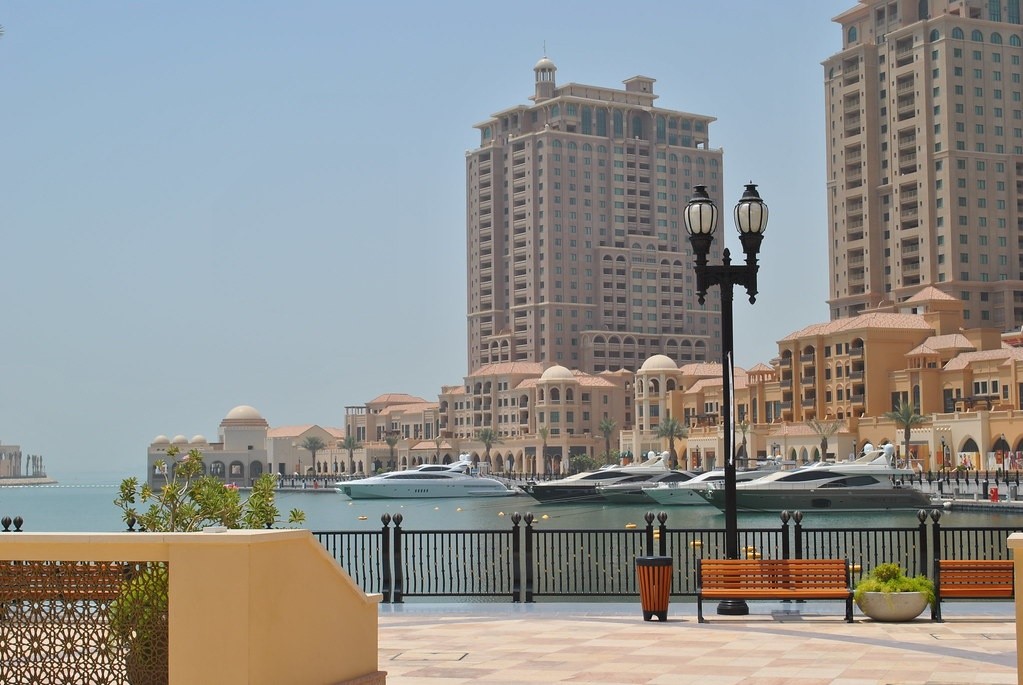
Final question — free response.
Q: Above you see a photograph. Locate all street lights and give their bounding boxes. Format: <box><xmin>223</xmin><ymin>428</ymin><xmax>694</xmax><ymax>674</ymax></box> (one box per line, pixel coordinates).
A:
<box><xmin>772</xmin><ymin>441</ymin><xmax>777</xmax><ymax>466</ymax></box>
<box><xmin>1001</xmin><ymin>433</ymin><xmax>1006</xmax><ymax>482</ymax></box>
<box><xmin>681</xmin><ymin>179</ymin><xmax>770</xmax><ymax>612</ymax></box>
<box><xmin>940</xmin><ymin>435</ymin><xmax>945</xmax><ymax>480</ymax></box>
<box><xmin>853</xmin><ymin>440</ymin><xmax>856</xmax><ymax>460</ymax></box>
<box><xmin>695</xmin><ymin>445</ymin><xmax>699</xmax><ymax>468</ymax></box>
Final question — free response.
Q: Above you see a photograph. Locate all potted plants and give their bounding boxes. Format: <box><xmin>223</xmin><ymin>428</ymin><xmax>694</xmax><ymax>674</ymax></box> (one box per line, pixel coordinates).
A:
<box><xmin>854</xmin><ymin>564</ymin><xmax>934</xmax><ymax>621</ymax></box>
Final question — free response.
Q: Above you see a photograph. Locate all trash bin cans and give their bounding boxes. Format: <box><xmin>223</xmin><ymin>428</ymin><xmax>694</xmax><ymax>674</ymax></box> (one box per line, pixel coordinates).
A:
<box><xmin>636</xmin><ymin>556</ymin><xmax>673</xmax><ymax>622</ymax></box>
<box><xmin>1010</xmin><ymin>486</ymin><xmax>1017</xmax><ymax>500</ymax></box>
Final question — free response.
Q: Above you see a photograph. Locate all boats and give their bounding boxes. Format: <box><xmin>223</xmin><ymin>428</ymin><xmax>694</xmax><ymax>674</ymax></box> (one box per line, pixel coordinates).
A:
<box><xmin>333</xmin><ymin>451</ymin><xmax>519</xmax><ymax>501</ymax></box>
<box><xmin>514</xmin><ymin>440</ymin><xmax>953</xmax><ymax>515</ymax></box>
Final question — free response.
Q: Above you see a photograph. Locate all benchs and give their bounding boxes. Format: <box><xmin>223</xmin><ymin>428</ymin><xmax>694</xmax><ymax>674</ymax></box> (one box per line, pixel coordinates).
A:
<box><xmin>695</xmin><ymin>558</ymin><xmax>854</xmax><ymax>623</ymax></box>
<box><xmin>933</xmin><ymin>558</ymin><xmax>1015</xmax><ymax>623</ymax></box>
<box><xmin>0</xmin><ymin>563</ymin><xmax>128</xmax><ymax>611</ymax></box>
